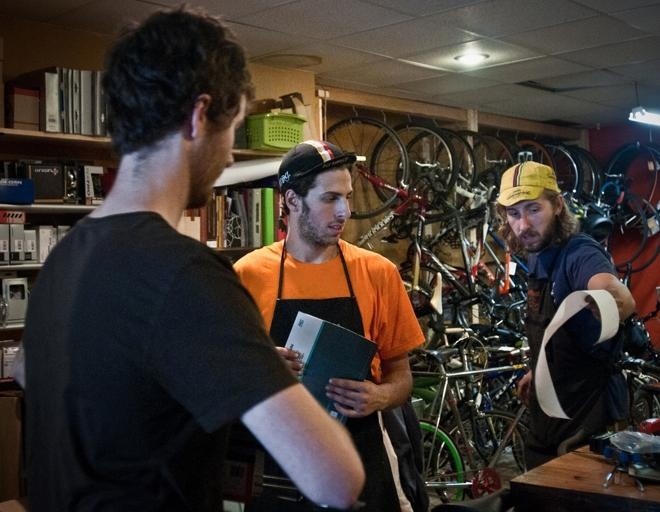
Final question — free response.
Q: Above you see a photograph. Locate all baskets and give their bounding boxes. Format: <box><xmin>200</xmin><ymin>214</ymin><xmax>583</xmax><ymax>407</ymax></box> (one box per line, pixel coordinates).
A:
<box><xmin>237</xmin><ymin>114</ymin><xmax>308</xmax><ymax>150</ymax></box>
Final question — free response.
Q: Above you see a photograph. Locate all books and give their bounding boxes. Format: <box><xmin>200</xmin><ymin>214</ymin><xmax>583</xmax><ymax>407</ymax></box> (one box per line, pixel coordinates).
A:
<box><xmin>31</xmin><ymin>65</ymin><xmax>112</xmax><ymax>138</ymax></box>
<box><xmin>280</xmin><ymin>308</ymin><xmax>380</xmax><ymax>439</ymax></box>
<box><xmin>181</xmin><ymin>185</ymin><xmax>291</xmax><ymax>250</ymax></box>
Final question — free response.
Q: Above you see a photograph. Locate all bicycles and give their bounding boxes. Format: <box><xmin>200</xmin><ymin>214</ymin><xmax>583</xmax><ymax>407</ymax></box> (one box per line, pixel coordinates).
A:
<box><xmin>326</xmin><ymin>115</ymin><xmax>660</xmax><ymax>503</ymax></box>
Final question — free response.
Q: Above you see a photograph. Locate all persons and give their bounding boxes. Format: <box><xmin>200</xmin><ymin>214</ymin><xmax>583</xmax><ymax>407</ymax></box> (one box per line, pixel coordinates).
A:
<box><xmin>229</xmin><ymin>139</ymin><xmax>428</xmax><ymax>510</ymax></box>
<box><xmin>19</xmin><ymin>7</ymin><xmax>372</xmax><ymax>512</ymax></box>
<box><xmin>493</xmin><ymin>159</ymin><xmax>638</xmax><ymax>476</ymax></box>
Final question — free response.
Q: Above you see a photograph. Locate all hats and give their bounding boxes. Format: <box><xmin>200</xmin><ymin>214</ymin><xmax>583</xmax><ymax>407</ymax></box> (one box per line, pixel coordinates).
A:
<box><xmin>497</xmin><ymin>160</ymin><xmax>561</xmax><ymax>207</ymax></box>
<box><xmin>279</xmin><ymin>139</ymin><xmax>358</xmax><ymax>193</ymax></box>
